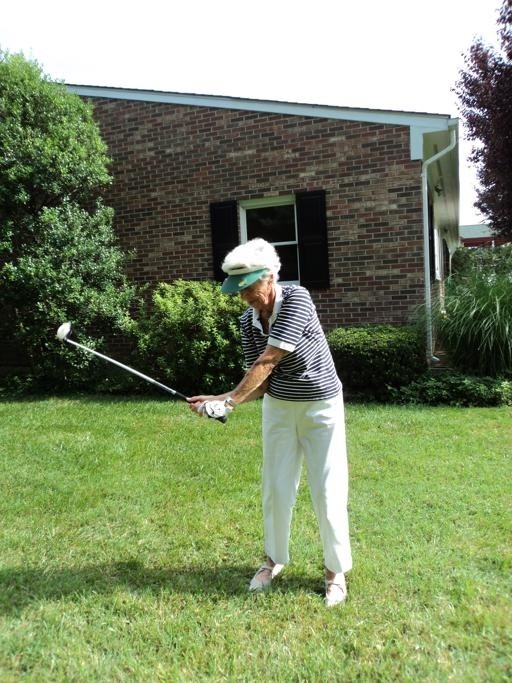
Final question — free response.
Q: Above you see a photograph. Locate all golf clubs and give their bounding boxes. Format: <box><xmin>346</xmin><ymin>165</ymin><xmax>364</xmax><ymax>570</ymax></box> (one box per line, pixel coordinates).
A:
<box><xmin>56</xmin><ymin>321</ymin><xmax>227</xmax><ymax>423</ymax></box>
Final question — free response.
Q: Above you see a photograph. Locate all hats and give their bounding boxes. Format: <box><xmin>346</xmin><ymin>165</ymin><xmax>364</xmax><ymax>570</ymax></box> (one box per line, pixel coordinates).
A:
<box><xmin>219</xmin><ymin>237</ymin><xmax>281</xmax><ymax>294</ymax></box>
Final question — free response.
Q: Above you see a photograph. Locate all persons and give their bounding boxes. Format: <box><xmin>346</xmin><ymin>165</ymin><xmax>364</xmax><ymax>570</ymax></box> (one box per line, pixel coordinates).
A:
<box><xmin>184</xmin><ymin>234</ymin><xmax>357</xmax><ymax>608</ymax></box>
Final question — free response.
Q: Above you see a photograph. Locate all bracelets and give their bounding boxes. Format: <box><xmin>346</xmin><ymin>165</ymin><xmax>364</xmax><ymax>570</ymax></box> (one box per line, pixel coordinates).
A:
<box><xmin>225</xmin><ymin>395</ymin><xmax>237</xmax><ymax>408</ymax></box>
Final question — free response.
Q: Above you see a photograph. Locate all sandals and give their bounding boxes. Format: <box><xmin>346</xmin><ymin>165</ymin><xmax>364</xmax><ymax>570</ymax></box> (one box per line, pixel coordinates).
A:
<box><xmin>324</xmin><ymin>567</ymin><xmax>347</xmax><ymax>608</ymax></box>
<box><xmin>248</xmin><ymin>564</ymin><xmax>274</xmax><ymax>592</ymax></box>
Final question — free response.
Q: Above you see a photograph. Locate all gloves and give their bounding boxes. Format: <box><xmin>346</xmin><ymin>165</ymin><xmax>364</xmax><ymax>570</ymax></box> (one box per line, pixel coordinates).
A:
<box><xmin>197</xmin><ymin>399</ymin><xmax>233</xmax><ymax>420</ymax></box>
